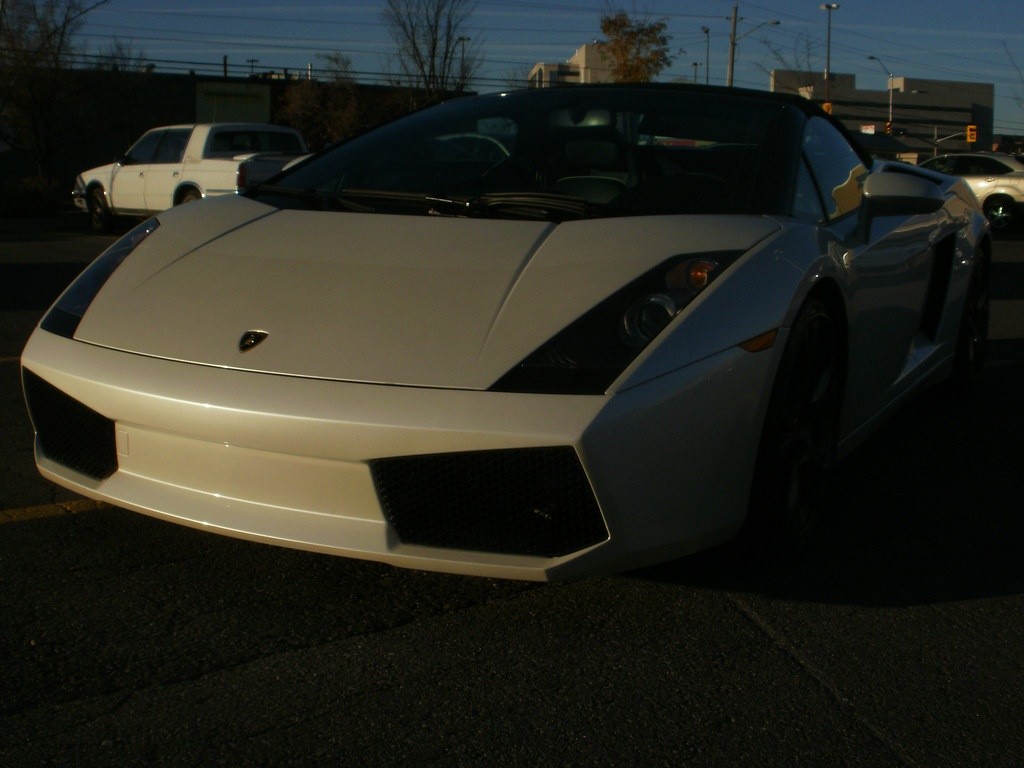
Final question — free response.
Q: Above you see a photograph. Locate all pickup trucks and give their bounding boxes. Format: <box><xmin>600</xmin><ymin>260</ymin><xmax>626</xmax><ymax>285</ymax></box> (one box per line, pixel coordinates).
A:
<box><xmin>70</xmin><ymin>121</ymin><xmax>314</xmax><ymax>230</ymax></box>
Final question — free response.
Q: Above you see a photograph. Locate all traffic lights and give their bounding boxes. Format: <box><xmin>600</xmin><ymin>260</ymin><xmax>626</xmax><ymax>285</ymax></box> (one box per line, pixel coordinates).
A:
<box><xmin>886</xmin><ymin>123</ymin><xmax>891</xmax><ymax>135</ymax></box>
<box><xmin>969</xmin><ymin>127</ymin><xmax>976</xmax><ymax>139</ymax></box>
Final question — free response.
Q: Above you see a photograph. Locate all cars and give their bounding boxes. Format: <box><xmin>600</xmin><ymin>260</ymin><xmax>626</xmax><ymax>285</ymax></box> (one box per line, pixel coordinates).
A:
<box><xmin>434</xmin><ymin>134</ymin><xmax>510</xmax><ymax>167</ymax></box>
<box><xmin>916</xmin><ymin>149</ymin><xmax>1024</xmax><ymax>234</ymax></box>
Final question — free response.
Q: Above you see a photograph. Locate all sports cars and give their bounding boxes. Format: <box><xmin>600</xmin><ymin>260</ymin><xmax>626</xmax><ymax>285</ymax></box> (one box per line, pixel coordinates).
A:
<box><xmin>17</xmin><ymin>79</ymin><xmax>996</xmax><ymax>587</ymax></box>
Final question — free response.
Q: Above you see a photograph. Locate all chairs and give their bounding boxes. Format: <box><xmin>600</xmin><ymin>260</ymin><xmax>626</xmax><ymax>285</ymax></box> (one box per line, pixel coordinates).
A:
<box><xmin>534</xmin><ymin>127</ymin><xmax>630</xmax><ymax>203</ymax></box>
<box><xmin>672</xmin><ymin>139</ymin><xmax>780</xmax><ymax>218</ymax></box>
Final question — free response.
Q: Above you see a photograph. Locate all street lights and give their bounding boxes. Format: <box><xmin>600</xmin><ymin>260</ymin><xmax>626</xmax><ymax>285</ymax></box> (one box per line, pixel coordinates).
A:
<box><xmin>246</xmin><ymin>57</ymin><xmax>259</xmax><ymax>72</ymax></box>
<box><xmin>458</xmin><ymin>35</ymin><xmax>471</xmax><ymax>89</ymax></box>
<box><xmin>702</xmin><ymin>26</ymin><xmax>710</xmax><ymax>85</ymax></box>
<box><xmin>819</xmin><ymin>2</ymin><xmax>840</xmax><ymax>101</ymax></box>
<box><xmin>868</xmin><ymin>56</ymin><xmax>893</xmax><ymax>122</ymax></box>
<box><xmin>693</xmin><ymin>62</ymin><xmax>702</xmax><ymax>83</ymax></box>
<box><xmin>726</xmin><ymin>19</ymin><xmax>781</xmax><ymax>87</ymax></box>
